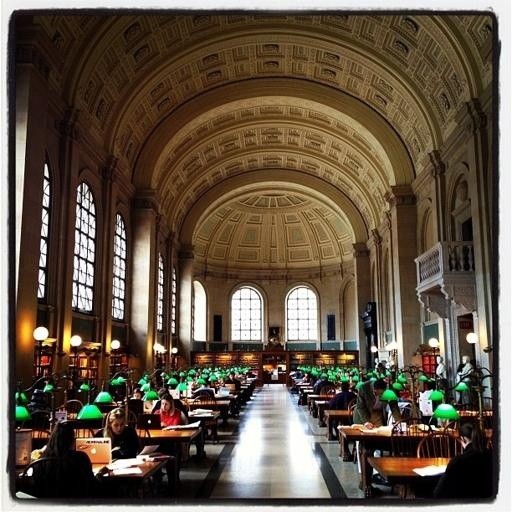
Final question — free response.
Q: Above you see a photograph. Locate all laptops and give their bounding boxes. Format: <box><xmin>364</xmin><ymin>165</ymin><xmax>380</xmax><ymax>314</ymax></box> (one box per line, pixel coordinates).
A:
<box><xmin>76</xmin><ymin>437</ymin><xmax>113</xmax><ymax>465</ymax></box>
<box><xmin>139</xmin><ymin>413</ymin><xmax>162</xmax><ymax>429</ymax></box>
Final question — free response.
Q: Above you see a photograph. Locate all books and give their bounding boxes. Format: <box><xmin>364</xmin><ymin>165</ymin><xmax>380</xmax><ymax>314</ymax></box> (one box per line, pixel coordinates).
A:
<box><xmin>35</xmin><ymin>356</ymin><xmax>99</xmax><ymax>386</ymax></box>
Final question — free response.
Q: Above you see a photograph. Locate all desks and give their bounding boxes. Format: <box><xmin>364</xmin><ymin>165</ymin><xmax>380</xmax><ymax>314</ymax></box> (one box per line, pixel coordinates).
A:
<box><xmin>292</xmin><ymin>378</ymin><xmax>494</xmax><ymax>501</ymax></box>
<box><xmin>14</xmin><ymin>377</ymin><xmax>257</xmax><ymax>499</ymax></box>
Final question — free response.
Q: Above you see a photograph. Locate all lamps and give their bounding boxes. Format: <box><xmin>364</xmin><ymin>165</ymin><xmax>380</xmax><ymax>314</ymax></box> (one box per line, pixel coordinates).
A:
<box><xmin>15</xmin><ymin>325</ymin><xmax>252</xmax><ymax>436</ymax></box>
<box><xmin>297</xmin><ymin>332</ymin><xmax>480</xmax><ymax>421</ymax></box>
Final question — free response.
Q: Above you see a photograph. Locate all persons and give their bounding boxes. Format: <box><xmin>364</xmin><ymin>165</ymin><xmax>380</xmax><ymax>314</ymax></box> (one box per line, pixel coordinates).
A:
<box><xmin>460</xmin><ymin>354</ymin><xmax>474</xmax><ymax>405</ymax></box>
<box><xmin>20</xmin><ymin>365</ymin><xmax>252</xmax><ymax>495</ymax></box>
<box><xmin>290</xmin><ymin>362</ymin><xmax>494</xmax><ymax>499</ymax></box>
<box><xmin>435</xmin><ymin>356</ymin><xmax>446</xmax><ymax>379</ymax></box>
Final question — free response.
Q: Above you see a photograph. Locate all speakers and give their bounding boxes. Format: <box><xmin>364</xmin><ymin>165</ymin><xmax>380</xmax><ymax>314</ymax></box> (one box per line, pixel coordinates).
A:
<box><xmin>326</xmin><ymin>314</ymin><xmax>336</xmax><ymax>340</ymax></box>
<box><xmin>213</xmin><ymin>314</ymin><xmax>223</xmax><ymax>342</ymax></box>
